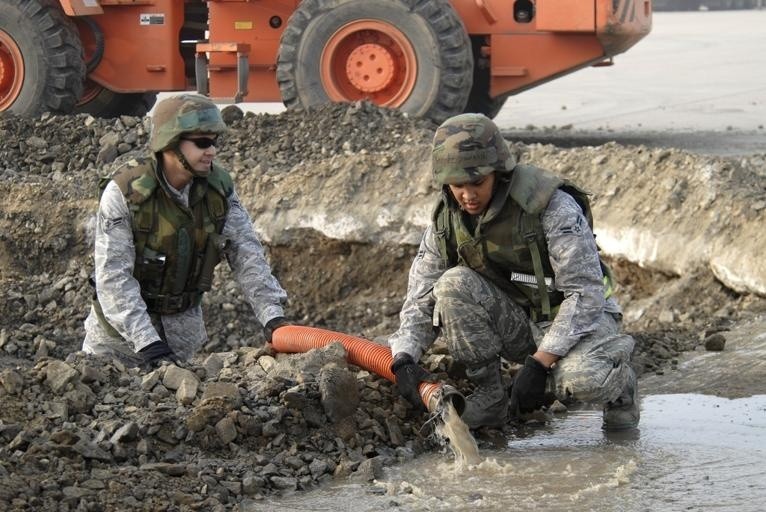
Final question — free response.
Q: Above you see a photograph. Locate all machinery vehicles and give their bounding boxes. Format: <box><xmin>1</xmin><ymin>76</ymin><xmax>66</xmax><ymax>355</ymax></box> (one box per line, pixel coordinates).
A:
<box><xmin>0</xmin><ymin>0</ymin><xmax>652</xmax><ymax>134</ymax></box>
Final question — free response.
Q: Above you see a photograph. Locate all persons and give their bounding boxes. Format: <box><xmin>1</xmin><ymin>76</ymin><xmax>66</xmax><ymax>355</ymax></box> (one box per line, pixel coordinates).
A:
<box><xmin>77</xmin><ymin>92</ymin><xmax>293</xmax><ymax>370</ymax></box>
<box><xmin>388</xmin><ymin>114</ymin><xmax>644</xmax><ymax>434</ymax></box>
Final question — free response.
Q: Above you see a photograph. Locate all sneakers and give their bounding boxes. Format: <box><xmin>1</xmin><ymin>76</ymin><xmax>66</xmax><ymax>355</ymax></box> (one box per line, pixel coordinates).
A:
<box><xmin>454</xmin><ymin>359</ymin><xmax>508</xmax><ymax>426</ymax></box>
<box><xmin>602</xmin><ymin>366</ymin><xmax>641</xmax><ymax>430</ymax></box>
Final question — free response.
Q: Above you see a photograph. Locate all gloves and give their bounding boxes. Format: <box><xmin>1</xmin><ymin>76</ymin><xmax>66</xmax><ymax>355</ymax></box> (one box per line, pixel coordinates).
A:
<box><xmin>509</xmin><ymin>357</ymin><xmax>549</xmax><ymax>418</ymax></box>
<box><xmin>389</xmin><ymin>352</ymin><xmax>439</xmax><ymax>413</ymax></box>
<box><xmin>142</xmin><ymin>341</ymin><xmax>183</xmax><ymax>374</ymax></box>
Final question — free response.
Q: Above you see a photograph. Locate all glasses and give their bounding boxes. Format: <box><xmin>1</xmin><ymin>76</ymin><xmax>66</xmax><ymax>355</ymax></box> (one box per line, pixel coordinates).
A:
<box><xmin>178</xmin><ymin>136</ymin><xmax>218</xmax><ymax>148</ymax></box>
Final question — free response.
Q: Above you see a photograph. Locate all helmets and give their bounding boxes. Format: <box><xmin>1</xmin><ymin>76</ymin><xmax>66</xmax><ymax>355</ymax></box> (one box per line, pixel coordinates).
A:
<box><xmin>150</xmin><ymin>94</ymin><xmax>227</xmax><ymax>153</ymax></box>
<box><xmin>431</xmin><ymin>112</ymin><xmax>515</xmax><ymax>185</ymax></box>
